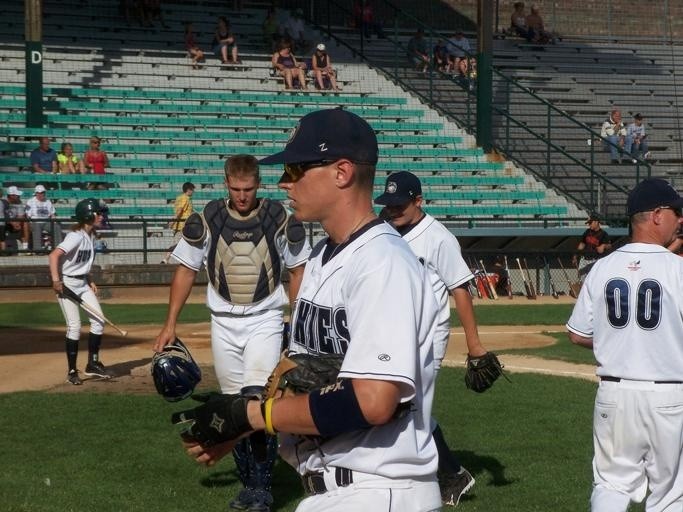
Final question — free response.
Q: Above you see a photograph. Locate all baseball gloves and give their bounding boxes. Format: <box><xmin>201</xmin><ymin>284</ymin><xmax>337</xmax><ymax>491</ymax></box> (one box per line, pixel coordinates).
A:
<box><xmin>263</xmin><ymin>349</ymin><xmax>414</xmax><ymax>450</ymax></box>
<box><xmin>464</xmin><ymin>351</ymin><xmax>501</xmax><ymax>393</ymax></box>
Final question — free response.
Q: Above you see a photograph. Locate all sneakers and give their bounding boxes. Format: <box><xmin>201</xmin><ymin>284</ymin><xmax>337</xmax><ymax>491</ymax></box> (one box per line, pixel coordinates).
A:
<box><xmin>248</xmin><ymin>488</ymin><xmax>273</xmax><ymax>512</ymax></box>
<box><xmin>83</xmin><ymin>360</ymin><xmax>113</xmax><ymax>378</ymax></box>
<box><xmin>66</xmin><ymin>371</ymin><xmax>82</xmax><ymax>386</ymax></box>
<box><xmin>229</xmin><ymin>485</ymin><xmax>253</xmax><ymax>509</ymax></box>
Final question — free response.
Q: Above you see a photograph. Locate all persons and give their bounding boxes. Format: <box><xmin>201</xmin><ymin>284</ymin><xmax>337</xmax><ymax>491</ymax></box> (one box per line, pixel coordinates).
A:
<box><xmin>183</xmin><ymin>24</ymin><xmax>204</xmax><ymax>64</ymax></box>
<box><xmin>0</xmin><ymin>186</ymin><xmax>31</xmax><ymax>255</ymax></box>
<box><xmin>527</xmin><ymin>6</ymin><xmax>546</xmax><ymax>43</ymax></box>
<box><xmin>212</xmin><ymin>16</ymin><xmax>241</xmax><ymax>63</ymax></box>
<box><xmin>510</xmin><ymin>2</ymin><xmax>539</xmax><ymax>41</ymax></box>
<box><xmin>628</xmin><ymin>112</ymin><xmax>653</xmax><ymax>164</ymax></box>
<box><xmin>311</xmin><ymin>44</ymin><xmax>341</xmax><ymax>89</ymax></box>
<box><xmin>58</xmin><ymin>142</ymin><xmax>86</xmax><ymax>174</ymax></box>
<box><xmin>49</xmin><ymin>198</ymin><xmax>114</xmax><ymax>387</ymax></box>
<box><xmin>373</xmin><ymin>172</ymin><xmax>502</xmax><ymax>507</ymax></box>
<box><xmin>458</xmin><ymin>54</ymin><xmax>477</xmax><ymax>80</ymax></box>
<box><xmin>162</xmin><ymin>180</ymin><xmax>196</xmax><ymax>266</ymax></box>
<box><xmin>260</xmin><ymin>8</ymin><xmax>308</xmax><ymax>49</ymax></box>
<box><xmin>433</xmin><ymin>38</ymin><xmax>453</xmax><ymax>71</ymax></box>
<box><xmin>567</xmin><ymin>176</ymin><xmax>683</xmax><ymax>512</ymax></box>
<box><xmin>84</xmin><ymin>136</ymin><xmax>113</xmax><ymax>204</ymax></box>
<box><xmin>447</xmin><ymin>30</ymin><xmax>471</xmax><ymax>64</ymax></box>
<box><xmin>489</xmin><ymin>252</ymin><xmax>509</xmax><ymax>297</ymax></box>
<box><xmin>173</xmin><ymin>106</ymin><xmax>446</xmax><ymax>512</ymax></box>
<box><xmin>407</xmin><ymin>27</ymin><xmax>433</xmax><ymax>73</ymax></box>
<box><xmin>664</xmin><ymin>196</ymin><xmax>683</xmax><ymax>260</ymax></box>
<box><xmin>153</xmin><ymin>153</ymin><xmax>312</xmax><ymax>512</ymax></box>
<box><xmin>600</xmin><ymin>110</ymin><xmax>633</xmax><ymax>165</ymax></box>
<box><xmin>31</xmin><ymin>137</ymin><xmax>58</xmax><ymax>190</ymax></box>
<box><xmin>571</xmin><ymin>213</ymin><xmax>613</xmax><ymax>287</ymax></box>
<box><xmin>26</xmin><ymin>185</ymin><xmax>58</xmax><ymax>250</ymax></box>
<box><xmin>270</xmin><ymin>42</ymin><xmax>307</xmax><ymax>89</ymax></box>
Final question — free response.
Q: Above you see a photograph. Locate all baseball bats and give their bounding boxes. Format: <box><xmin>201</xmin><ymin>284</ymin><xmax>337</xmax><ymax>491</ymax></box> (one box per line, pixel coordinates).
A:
<box><xmin>62</xmin><ymin>285</ymin><xmax>127</xmax><ymax>337</ymax></box>
<box><xmin>503</xmin><ymin>255</ymin><xmax>512</xmax><ymax>300</ymax></box>
<box><xmin>556</xmin><ymin>256</ymin><xmax>577</xmax><ymax>299</ymax></box>
<box><xmin>467</xmin><ymin>255</ymin><xmax>498</xmax><ymax>300</ymax></box>
<box><xmin>542</xmin><ymin>255</ymin><xmax>559</xmax><ymax>299</ymax></box>
<box><xmin>515</xmin><ymin>257</ymin><xmax>531</xmax><ymax>297</ymax></box>
<box><xmin>523</xmin><ymin>258</ymin><xmax>534</xmax><ymax>297</ymax></box>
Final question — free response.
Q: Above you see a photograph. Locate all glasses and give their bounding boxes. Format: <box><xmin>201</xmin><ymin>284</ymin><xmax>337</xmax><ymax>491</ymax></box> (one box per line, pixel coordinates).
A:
<box><xmin>90</xmin><ymin>140</ymin><xmax>102</xmax><ymax>145</ymax></box>
<box><xmin>279</xmin><ymin>162</ymin><xmax>333</xmax><ymax>179</ymax></box>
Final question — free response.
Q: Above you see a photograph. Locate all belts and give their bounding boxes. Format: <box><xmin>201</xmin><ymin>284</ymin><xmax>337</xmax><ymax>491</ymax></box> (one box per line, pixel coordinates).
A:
<box><xmin>67</xmin><ymin>275</ymin><xmax>88</xmax><ymax>279</ymax></box>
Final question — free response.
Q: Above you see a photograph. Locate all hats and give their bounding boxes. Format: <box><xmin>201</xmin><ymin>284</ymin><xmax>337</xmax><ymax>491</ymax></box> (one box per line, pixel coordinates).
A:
<box><xmin>33</xmin><ymin>184</ymin><xmax>46</xmax><ymax>193</ymax></box>
<box><xmin>634</xmin><ymin>112</ymin><xmax>644</xmax><ymax>120</ymax></box>
<box><xmin>374</xmin><ymin>170</ymin><xmax>423</xmax><ymax>205</ymax></box>
<box><xmin>6</xmin><ymin>186</ymin><xmax>24</xmax><ymax>196</ymax></box>
<box><xmin>317</xmin><ymin>43</ymin><xmax>325</xmax><ymax>52</ymax></box>
<box><xmin>585</xmin><ymin>212</ymin><xmax>602</xmax><ymax>223</ymax></box>
<box><xmin>257</xmin><ymin>109</ymin><xmax>378</xmax><ymax>165</ymax></box>
<box><xmin>626</xmin><ymin>178</ymin><xmax>683</xmax><ymax>217</ymax></box>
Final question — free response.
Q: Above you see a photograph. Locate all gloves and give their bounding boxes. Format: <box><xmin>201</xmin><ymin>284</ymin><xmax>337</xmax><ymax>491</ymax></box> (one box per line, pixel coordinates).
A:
<box><xmin>171</xmin><ymin>391</ymin><xmax>253</xmax><ymax>444</ymax></box>
<box><xmin>466</xmin><ymin>351</ymin><xmax>498</xmax><ymax>392</ymax></box>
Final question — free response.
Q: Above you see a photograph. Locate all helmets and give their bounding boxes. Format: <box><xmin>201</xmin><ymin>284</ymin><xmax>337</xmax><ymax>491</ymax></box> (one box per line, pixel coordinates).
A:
<box><xmin>75</xmin><ymin>198</ymin><xmax>110</xmax><ymax>225</ymax></box>
<box><xmin>154</xmin><ymin>340</ymin><xmax>200</xmax><ymax>401</ymax></box>
